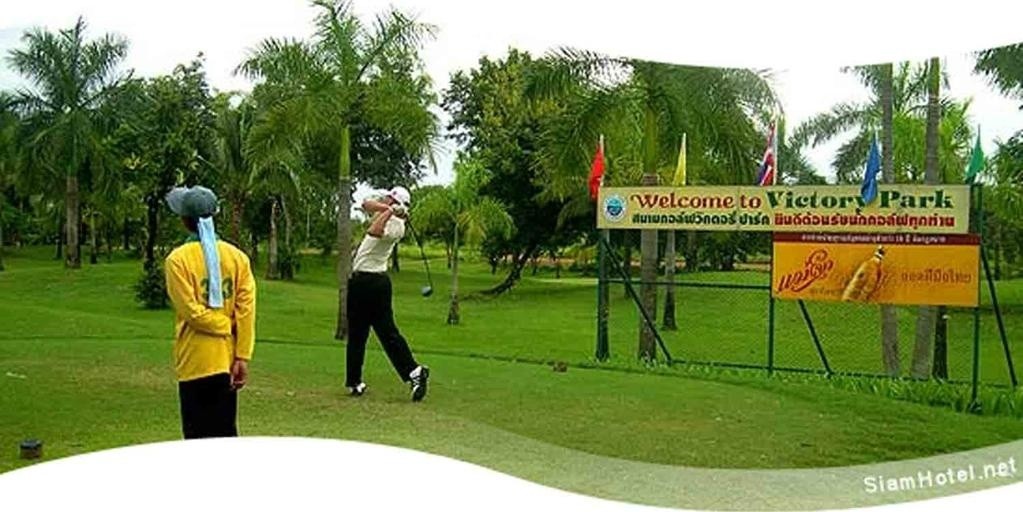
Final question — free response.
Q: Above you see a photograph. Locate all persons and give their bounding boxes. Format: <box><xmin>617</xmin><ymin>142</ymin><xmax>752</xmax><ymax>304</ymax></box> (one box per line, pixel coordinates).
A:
<box><xmin>340</xmin><ymin>183</ymin><xmax>430</xmax><ymax>404</ymax></box>
<box><xmin>160</xmin><ymin>183</ymin><xmax>257</xmax><ymax>439</ymax></box>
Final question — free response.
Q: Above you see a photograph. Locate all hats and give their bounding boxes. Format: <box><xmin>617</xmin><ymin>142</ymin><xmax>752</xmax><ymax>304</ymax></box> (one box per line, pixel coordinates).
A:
<box><xmin>376</xmin><ymin>186</ymin><xmax>412</xmax><ymax>207</ymax></box>
<box><xmin>165</xmin><ymin>184</ymin><xmax>221</xmax><ymax>217</ymax></box>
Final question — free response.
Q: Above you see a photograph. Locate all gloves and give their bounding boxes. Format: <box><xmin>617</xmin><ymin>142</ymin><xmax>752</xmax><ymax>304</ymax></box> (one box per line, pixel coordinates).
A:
<box><xmin>387</xmin><ymin>201</ymin><xmax>411</xmax><ymax>219</ymax></box>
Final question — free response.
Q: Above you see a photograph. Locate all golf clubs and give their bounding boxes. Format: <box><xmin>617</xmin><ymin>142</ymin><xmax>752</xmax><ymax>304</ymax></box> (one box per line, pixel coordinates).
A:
<box><xmin>394</xmin><ymin>207</ymin><xmax>433</xmax><ymax>297</ymax></box>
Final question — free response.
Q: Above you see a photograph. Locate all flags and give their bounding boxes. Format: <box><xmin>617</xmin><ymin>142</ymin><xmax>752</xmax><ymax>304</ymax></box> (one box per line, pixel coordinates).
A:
<box><xmin>858</xmin><ymin>130</ymin><xmax>883</xmax><ymax>209</ymax></box>
<box><xmin>668</xmin><ymin>131</ymin><xmax>692</xmax><ymax>190</ymax></box>
<box><xmin>961</xmin><ymin>124</ymin><xmax>986</xmax><ymax>187</ymax></box>
<box><xmin>752</xmin><ymin>117</ymin><xmax>779</xmax><ymax>186</ymax></box>
<box><xmin>586</xmin><ymin>132</ymin><xmax>610</xmax><ymax>204</ymax></box>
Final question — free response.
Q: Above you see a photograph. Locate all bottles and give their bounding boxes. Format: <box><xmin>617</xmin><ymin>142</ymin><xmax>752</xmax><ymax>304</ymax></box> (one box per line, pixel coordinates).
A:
<box><xmin>841</xmin><ymin>242</ymin><xmax>886</xmax><ymax>305</ymax></box>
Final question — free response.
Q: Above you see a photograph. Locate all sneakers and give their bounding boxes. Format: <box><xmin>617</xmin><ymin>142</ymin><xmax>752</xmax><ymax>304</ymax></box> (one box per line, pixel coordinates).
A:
<box><xmin>349</xmin><ymin>381</ymin><xmax>366</xmax><ymax>397</ymax></box>
<box><xmin>409</xmin><ymin>364</ymin><xmax>431</xmax><ymax>402</ymax></box>
